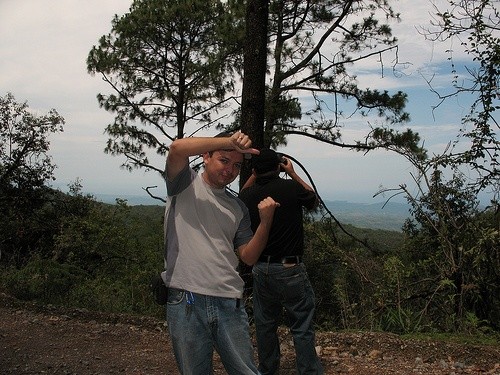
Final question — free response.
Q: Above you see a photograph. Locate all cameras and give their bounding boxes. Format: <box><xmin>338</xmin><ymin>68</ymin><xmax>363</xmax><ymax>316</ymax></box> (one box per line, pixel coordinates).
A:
<box><xmin>280</xmin><ymin>158</ymin><xmax>287</xmax><ymax>172</ymax></box>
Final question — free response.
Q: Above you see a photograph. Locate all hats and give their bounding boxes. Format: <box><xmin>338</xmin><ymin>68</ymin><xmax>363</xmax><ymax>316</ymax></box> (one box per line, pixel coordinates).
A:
<box><xmin>253</xmin><ymin>148</ymin><xmax>283</xmax><ymax>173</ymax></box>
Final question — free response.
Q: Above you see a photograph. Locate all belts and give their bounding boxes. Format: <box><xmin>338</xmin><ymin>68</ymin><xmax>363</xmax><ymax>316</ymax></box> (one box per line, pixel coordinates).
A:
<box><xmin>259</xmin><ymin>255</ymin><xmax>302</xmax><ymax>264</ymax></box>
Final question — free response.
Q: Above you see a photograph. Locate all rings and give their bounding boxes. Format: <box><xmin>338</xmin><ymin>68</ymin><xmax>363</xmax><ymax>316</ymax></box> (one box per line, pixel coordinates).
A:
<box><xmin>237</xmin><ymin>137</ymin><xmax>244</xmax><ymax>139</ymax></box>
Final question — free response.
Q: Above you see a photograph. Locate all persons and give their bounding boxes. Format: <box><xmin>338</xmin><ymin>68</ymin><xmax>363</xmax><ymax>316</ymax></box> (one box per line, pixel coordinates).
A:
<box><xmin>160</xmin><ymin>131</ymin><xmax>280</xmax><ymax>375</ymax></box>
<box><xmin>238</xmin><ymin>149</ymin><xmax>324</xmax><ymax>375</ymax></box>
